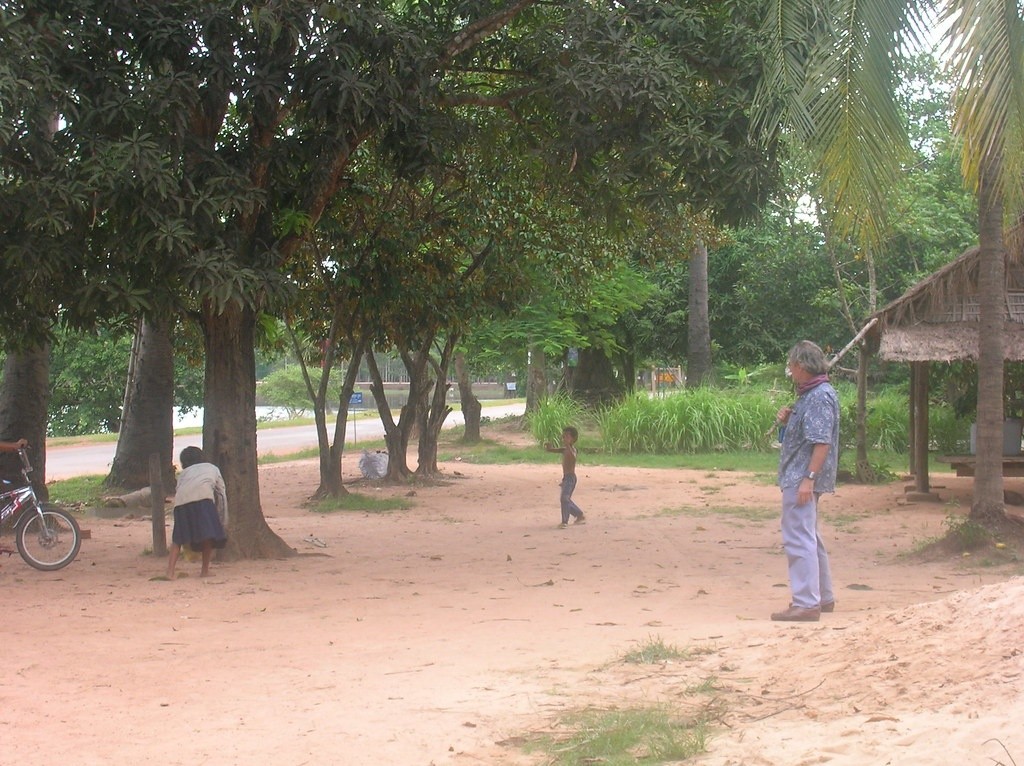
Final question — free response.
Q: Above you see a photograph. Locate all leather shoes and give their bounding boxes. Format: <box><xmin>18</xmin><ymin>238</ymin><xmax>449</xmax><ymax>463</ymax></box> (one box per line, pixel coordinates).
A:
<box><xmin>788</xmin><ymin>600</ymin><xmax>835</xmax><ymax>613</ymax></box>
<box><xmin>772</xmin><ymin>606</ymin><xmax>822</xmax><ymax>621</ymax></box>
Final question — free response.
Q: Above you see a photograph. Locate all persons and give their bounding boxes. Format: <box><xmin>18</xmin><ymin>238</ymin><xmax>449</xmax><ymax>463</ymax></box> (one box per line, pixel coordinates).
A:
<box><xmin>771</xmin><ymin>339</ymin><xmax>841</xmax><ymax>622</ymax></box>
<box><xmin>163</xmin><ymin>446</ymin><xmax>227</xmax><ymax>581</ymax></box>
<box><xmin>0</xmin><ymin>439</ymin><xmax>29</xmax><ymax>453</ymax></box>
<box><xmin>543</xmin><ymin>427</ymin><xmax>585</xmax><ymax>528</ymax></box>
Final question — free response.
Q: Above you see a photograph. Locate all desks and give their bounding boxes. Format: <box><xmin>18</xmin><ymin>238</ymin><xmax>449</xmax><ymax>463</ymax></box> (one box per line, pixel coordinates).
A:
<box><xmin>933</xmin><ymin>453</ymin><xmax>1024</xmax><ymax>476</ymax></box>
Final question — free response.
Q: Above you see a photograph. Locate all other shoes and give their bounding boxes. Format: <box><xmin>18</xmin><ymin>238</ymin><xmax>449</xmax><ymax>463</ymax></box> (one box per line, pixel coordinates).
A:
<box><xmin>573</xmin><ymin>515</ymin><xmax>586</xmax><ymax>524</ymax></box>
<box><xmin>557</xmin><ymin>523</ymin><xmax>567</xmax><ymax>529</ymax></box>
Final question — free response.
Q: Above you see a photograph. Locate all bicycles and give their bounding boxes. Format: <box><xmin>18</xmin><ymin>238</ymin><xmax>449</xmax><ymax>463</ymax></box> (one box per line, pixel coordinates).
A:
<box><xmin>0</xmin><ymin>445</ymin><xmax>83</xmax><ymax>571</ymax></box>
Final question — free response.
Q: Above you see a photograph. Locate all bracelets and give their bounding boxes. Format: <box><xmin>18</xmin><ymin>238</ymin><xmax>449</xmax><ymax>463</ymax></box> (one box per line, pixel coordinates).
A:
<box><xmin>805</xmin><ymin>470</ymin><xmax>818</xmax><ymax>480</ymax></box>
